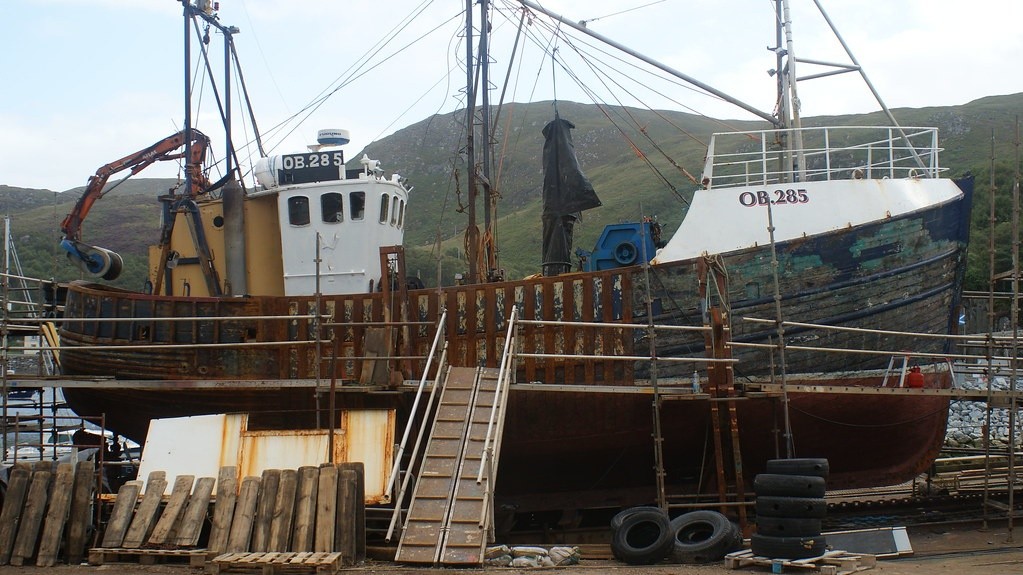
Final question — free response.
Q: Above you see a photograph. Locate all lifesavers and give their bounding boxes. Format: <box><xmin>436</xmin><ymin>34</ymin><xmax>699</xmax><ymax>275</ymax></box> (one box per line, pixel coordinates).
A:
<box><xmin>851</xmin><ymin>169</ymin><xmax>864</xmax><ymax>180</ymax></box>
<box><xmin>907</xmin><ymin>169</ymin><xmax>918</xmax><ymax>179</ymax></box>
<box><xmin>883</xmin><ymin>176</ymin><xmax>889</xmax><ymax>179</ymax></box>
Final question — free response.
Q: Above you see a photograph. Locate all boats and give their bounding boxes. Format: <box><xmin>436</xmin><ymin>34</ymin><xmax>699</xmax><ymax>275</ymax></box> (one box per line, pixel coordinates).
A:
<box><xmin>51</xmin><ymin>0</ymin><xmax>976</xmax><ymax>540</ymax></box>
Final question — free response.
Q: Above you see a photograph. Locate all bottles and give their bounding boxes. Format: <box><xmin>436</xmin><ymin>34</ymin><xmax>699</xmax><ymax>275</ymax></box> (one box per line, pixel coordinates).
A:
<box><xmin>7</xmin><ymin>354</ymin><xmax>40</xmax><ymax>375</ymax></box>
<box><xmin>693</xmin><ymin>371</ymin><xmax>700</xmax><ymax>394</ymax></box>
